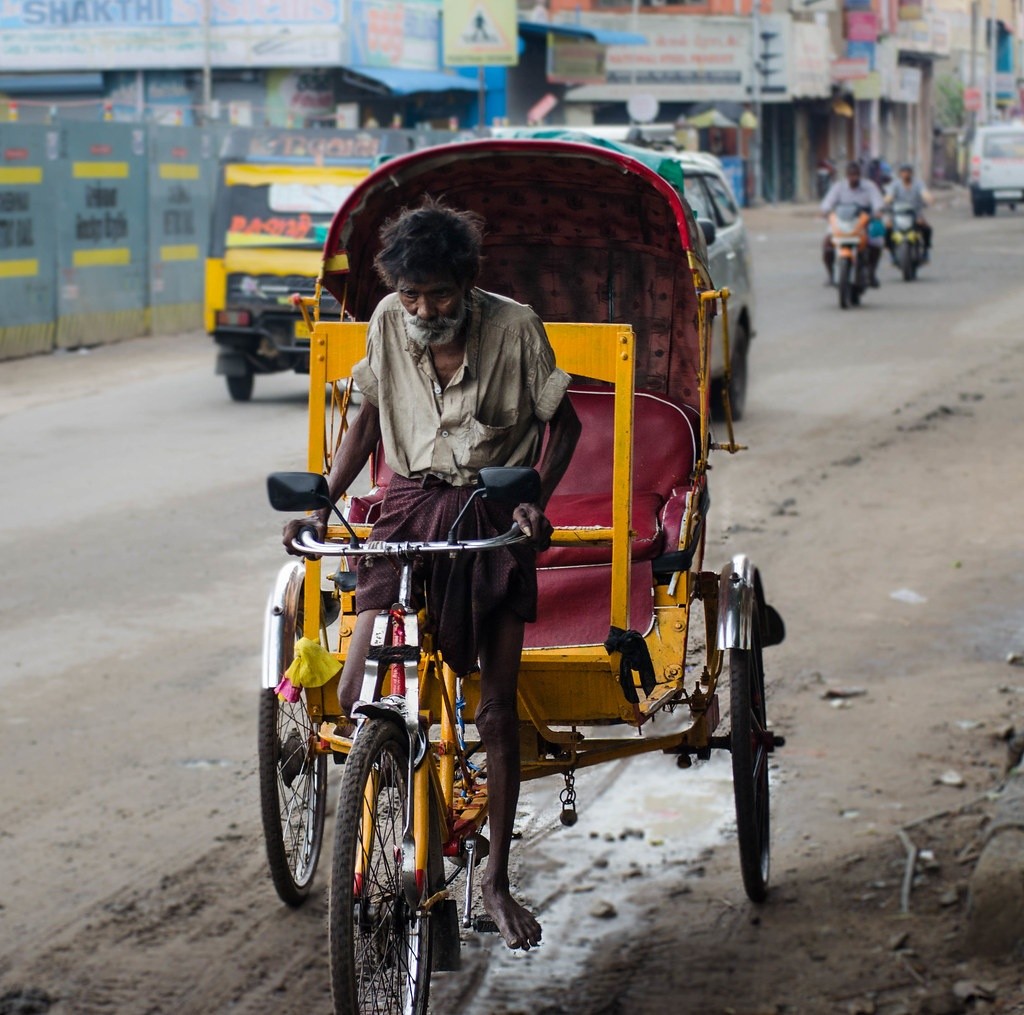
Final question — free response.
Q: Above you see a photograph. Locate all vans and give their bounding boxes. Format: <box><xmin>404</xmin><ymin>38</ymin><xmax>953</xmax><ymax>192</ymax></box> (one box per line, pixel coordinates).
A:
<box><xmin>968</xmin><ymin>125</ymin><xmax>1024</xmax><ymax>216</ymax></box>
<box><xmin>198</xmin><ymin>127</ymin><xmax>475</xmax><ymax>401</ymax></box>
<box><xmin>658</xmin><ymin>146</ymin><xmax>757</xmax><ymax>422</ymax></box>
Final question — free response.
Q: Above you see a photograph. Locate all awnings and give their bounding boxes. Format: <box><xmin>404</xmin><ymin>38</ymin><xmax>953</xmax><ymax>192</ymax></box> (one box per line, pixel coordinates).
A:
<box><xmin>519</xmin><ymin>17</ymin><xmax>647</xmax><ymax>45</ymax></box>
<box><xmin>339</xmin><ymin>62</ymin><xmax>489</xmax><ymax>101</ymax></box>
<box><xmin>564</xmin><ymin>80</ymin><xmax>756</xmax><ymax>108</ymax></box>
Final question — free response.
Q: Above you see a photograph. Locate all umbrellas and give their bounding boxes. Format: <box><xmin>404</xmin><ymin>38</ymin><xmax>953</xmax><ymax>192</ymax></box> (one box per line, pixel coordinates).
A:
<box><xmin>674</xmin><ymin>98</ymin><xmax>759</xmax><ymax>156</ymax></box>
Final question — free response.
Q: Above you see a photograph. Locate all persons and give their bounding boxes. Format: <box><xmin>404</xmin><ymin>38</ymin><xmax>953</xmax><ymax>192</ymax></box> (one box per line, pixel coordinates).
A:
<box><xmin>281</xmin><ymin>204</ymin><xmax>584</xmax><ymax>950</ymax></box>
<box><xmin>882</xmin><ymin>162</ymin><xmax>936</xmax><ymax>265</ymax></box>
<box><xmin>816</xmin><ymin>158</ymin><xmax>890</xmax><ymax>288</ymax></box>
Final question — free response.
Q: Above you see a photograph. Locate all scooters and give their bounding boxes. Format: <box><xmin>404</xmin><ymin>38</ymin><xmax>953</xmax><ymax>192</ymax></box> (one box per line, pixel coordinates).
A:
<box><xmin>876</xmin><ymin>197</ymin><xmax>931</xmax><ymax>283</ymax></box>
<box><xmin>822</xmin><ymin>201</ymin><xmax>874</xmax><ymax>310</ymax></box>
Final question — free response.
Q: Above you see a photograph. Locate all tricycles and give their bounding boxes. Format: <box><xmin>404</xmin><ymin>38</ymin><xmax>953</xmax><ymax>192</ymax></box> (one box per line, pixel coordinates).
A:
<box><xmin>253</xmin><ymin>137</ymin><xmax>789</xmax><ymax>1014</ymax></box>
